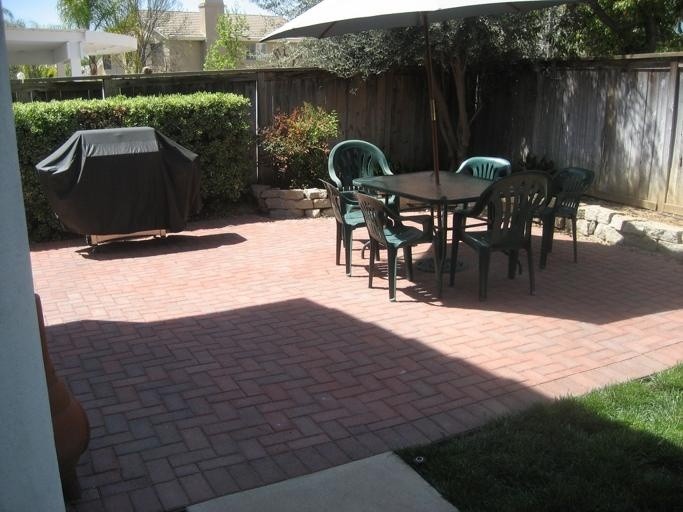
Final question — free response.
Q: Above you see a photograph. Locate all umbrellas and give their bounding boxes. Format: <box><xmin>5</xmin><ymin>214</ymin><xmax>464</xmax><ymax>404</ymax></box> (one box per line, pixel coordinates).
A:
<box><xmin>258</xmin><ymin>0</ymin><xmax>575</xmax><ymax>274</ymax></box>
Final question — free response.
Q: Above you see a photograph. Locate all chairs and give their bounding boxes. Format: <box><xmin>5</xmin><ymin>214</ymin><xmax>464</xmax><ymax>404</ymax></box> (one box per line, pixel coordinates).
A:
<box><xmin>355</xmin><ymin>192</ymin><xmax>440</xmax><ymax>303</ymax></box>
<box><xmin>323</xmin><ymin>139</ymin><xmax>396</xmax><ymax>205</ymax></box>
<box><xmin>320</xmin><ymin>178</ymin><xmax>396</xmax><ymax>277</ymax></box>
<box><xmin>456</xmin><ymin>155</ymin><xmax>511</xmax><ymax>185</ymax></box>
<box><xmin>450</xmin><ymin>171</ymin><xmax>548</xmax><ymax>299</ymax></box>
<box><xmin>538</xmin><ymin>167</ymin><xmax>583</xmax><ymax>271</ymax></box>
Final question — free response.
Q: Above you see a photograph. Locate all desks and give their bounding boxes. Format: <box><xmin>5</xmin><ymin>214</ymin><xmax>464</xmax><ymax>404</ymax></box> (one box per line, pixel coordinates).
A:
<box><xmin>353</xmin><ymin>169</ymin><xmax>532</xmax><ymax>276</ymax></box>
